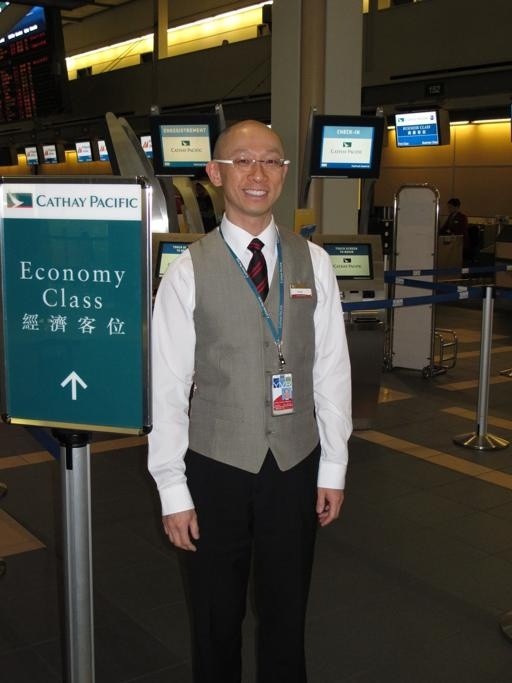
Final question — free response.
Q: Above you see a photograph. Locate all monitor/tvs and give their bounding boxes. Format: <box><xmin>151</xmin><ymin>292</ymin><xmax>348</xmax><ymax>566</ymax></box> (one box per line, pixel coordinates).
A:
<box><xmin>149</xmin><ymin>112</ymin><xmax>219</xmax><ymax>177</ymax></box>
<box><xmin>395</xmin><ymin>109</ymin><xmax>451</xmax><ymax>148</ymax></box>
<box><xmin>311</xmin><ymin>233</ymin><xmax>386</xmax><ymax>301</ymax></box>
<box><xmin>309</xmin><ymin>114</ymin><xmax>385</xmax><ymax>178</ymax></box>
<box><xmin>151</xmin><ymin>232</ymin><xmax>208</xmax><ymax>305</ymax></box>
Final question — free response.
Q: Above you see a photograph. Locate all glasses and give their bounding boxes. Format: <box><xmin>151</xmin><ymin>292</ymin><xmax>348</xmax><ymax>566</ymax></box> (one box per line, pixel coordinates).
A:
<box><xmin>213</xmin><ymin>155</ymin><xmax>291</xmax><ymax>169</ymax></box>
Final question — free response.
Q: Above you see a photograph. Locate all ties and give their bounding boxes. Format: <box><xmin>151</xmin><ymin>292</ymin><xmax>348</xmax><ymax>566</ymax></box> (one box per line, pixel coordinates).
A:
<box><xmin>246</xmin><ymin>238</ymin><xmax>269</xmax><ymax>303</ymax></box>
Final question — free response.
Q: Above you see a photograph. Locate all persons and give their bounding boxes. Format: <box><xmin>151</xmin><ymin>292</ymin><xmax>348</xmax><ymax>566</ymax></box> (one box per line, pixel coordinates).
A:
<box><xmin>147</xmin><ymin>118</ymin><xmax>353</xmax><ymax>682</ymax></box>
